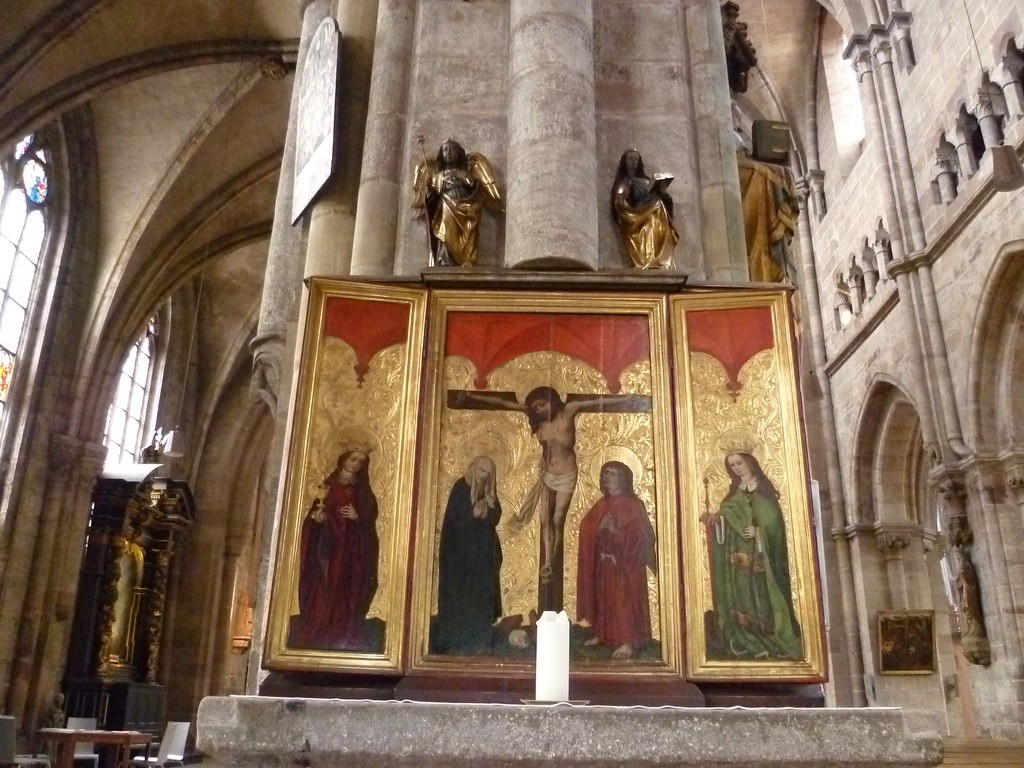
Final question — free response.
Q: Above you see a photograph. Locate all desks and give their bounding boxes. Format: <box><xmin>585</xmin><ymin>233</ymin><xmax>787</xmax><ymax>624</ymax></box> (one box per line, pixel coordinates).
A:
<box><xmin>38</xmin><ymin>729</ymin><xmax>153</xmax><ymax>768</ymax></box>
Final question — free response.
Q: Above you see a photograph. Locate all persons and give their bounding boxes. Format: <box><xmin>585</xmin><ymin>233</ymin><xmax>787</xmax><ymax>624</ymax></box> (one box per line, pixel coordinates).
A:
<box><xmin>612</xmin><ymin>148</ymin><xmax>680</xmax><ymax>270</ymax></box>
<box><xmin>422</xmin><ymin>136</ymin><xmax>485</xmax><ymax>268</ymax></box>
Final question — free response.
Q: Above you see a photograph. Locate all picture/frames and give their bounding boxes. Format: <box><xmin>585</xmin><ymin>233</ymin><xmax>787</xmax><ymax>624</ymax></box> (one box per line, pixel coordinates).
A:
<box><xmin>254</xmin><ymin>275</ymin><xmax>427</xmax><ymax>675</ymax></box>
<box><xmin>405</xmin><ymin>284</ymin><xmax>685</xmax><ymax>682</ymax></box>
<box><xmin>669</xmin><ymin>287</ymin><xmax>831</xmax><ymax>685</ymax></box>
<box><xmin>876</xmin><ymin>609</ymin><xmax>938</xmax><ymax>676</ymax></box>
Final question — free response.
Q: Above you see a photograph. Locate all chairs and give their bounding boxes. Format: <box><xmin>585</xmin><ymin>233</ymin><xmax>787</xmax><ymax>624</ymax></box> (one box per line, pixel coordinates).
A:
<box><xmin>133</xmin><ymin>720</ymin><xmax>191</xmax><ymax>768</ymax></box>
<box><xmin>64</xmin><ymin>718</ymin><xmax>99</xmax><ymax>768</ymax></box>
<box><xmin>0</xmin><ymin>714</ymin><xmax>52</xmax><ymax>768</ymax></box>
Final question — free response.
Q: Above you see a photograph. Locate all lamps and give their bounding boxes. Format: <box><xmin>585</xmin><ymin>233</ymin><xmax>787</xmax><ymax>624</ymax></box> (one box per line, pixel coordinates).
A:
<box><xmin>160</xmin><ymin>0</ymin><xmax>254</xmax><ymax>459</ymax></box>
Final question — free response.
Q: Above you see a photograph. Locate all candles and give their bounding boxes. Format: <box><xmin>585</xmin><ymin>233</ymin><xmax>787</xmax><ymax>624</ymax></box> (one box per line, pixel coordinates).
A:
<box><xmin>535</xmin><ymin>609</ymin><xmax>569</xmax><ymax>701</ymax></box>
